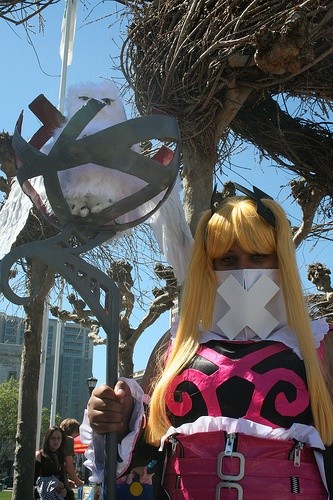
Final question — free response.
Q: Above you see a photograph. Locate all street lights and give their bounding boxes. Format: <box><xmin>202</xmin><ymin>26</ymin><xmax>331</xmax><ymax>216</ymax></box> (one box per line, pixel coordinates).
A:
<box><xmin>83</xmin><ymin>376</ymin><xmax>98</xmax><ymax>484</ymax></box>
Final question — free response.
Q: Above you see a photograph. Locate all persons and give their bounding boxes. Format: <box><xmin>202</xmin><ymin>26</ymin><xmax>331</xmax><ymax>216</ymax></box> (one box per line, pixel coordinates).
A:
<box><xmin>59</xmin><ymin>418</ymin><xmax>84</xmax><ymax>500</ymax></box>
<box><xmin>33</xmin><ymin>426</ymin><xmax>74</xmax><ymax>500</ymax></box>
<box><xmin>79</xmin><ymin>182</ymin><xmax>333</xmax><ymax>500</ymax></box>
